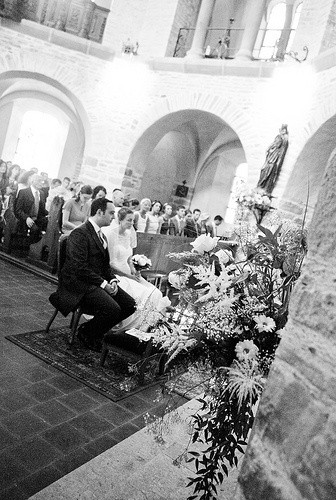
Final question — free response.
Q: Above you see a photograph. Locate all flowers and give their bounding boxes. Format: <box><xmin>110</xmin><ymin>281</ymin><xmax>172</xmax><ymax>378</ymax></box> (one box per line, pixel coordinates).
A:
<box><xmin>123</xmin><ymin>166</ymin><xmax>316</xmax><ymax>500</ymax></box>
<box><xmin>131</xmin><ymin>253</ymin><xmax>154</xmax><ymax>276</ymax></box>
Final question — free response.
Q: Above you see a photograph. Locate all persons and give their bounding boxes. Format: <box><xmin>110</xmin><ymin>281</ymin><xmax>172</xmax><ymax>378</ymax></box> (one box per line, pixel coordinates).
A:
<box><xmin>55</xmin><ymin>199</ymin><xmax>137</xmax><ymax>352</ymax></box>
<box><xmin>112</xmin><ymin>189</ymin><xmax>224</xmax><ymax>239</ymax></box>
<box><xmin>106</xmin><ymin>207</ymin><xmax>163</xmax><ymax>341</ymax></box>
<box><xmin>0</xmin><ymin>159</ymin><xmax>108</xmax><ymax>261</ymax></box>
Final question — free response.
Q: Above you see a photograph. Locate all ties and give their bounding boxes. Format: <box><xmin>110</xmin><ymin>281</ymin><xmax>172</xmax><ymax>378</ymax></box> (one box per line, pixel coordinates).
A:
<box><xmin>99</xmin><ymin>232</ymin><xmax>104</xmax><ymax>245</ymax></box>
<box><xmin>35</xmin><ymin>191</ymin><xmax>39</xmax><ymax>215</ymax></box>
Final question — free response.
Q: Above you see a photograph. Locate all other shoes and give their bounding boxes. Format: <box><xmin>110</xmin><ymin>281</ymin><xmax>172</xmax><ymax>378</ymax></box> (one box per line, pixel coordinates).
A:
<box><xmin>77</xmin><ymin>331</ymin><xmax>103</xmax><ymax>353</ymax></box>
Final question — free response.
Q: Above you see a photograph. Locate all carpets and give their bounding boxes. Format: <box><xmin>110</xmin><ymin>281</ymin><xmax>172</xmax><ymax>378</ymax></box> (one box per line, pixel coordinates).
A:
<box><xmin>4</xmin><ymin>322</ymin><xmax>186</xmax><ymax>403</ymax></box>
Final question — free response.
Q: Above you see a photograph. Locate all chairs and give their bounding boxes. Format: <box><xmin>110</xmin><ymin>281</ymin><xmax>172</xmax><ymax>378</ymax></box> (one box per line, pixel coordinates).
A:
<box><xmin>45</xmin><ymin>231</ymin><xmax>81</xmax><ymax>346</ymax></box>
